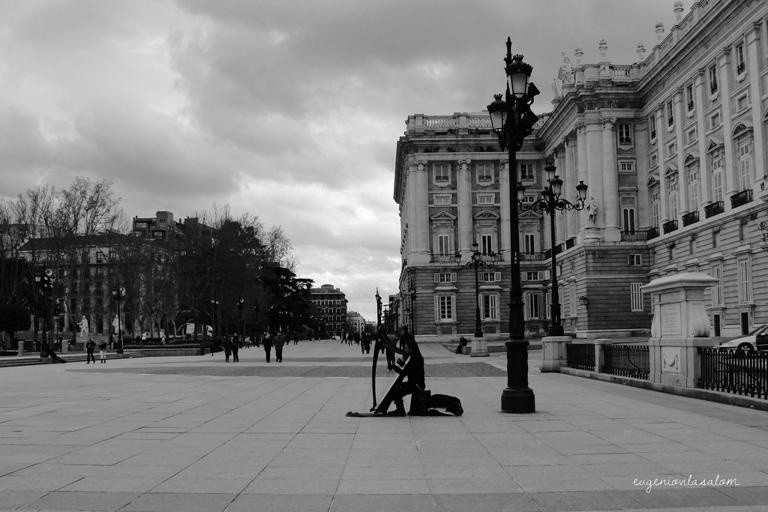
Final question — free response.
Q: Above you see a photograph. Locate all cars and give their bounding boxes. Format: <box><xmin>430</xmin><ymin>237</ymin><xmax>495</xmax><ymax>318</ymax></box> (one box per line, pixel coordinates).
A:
<box><xmin>721</xmin><ymin>321</ymin><xmax>768</xmax><ymax>355</ymax></box>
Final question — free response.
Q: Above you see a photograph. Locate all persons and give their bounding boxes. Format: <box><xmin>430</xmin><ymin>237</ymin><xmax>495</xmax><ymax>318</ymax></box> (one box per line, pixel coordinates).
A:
<box><xmin>84</xmin><ymin>338</ymin><xmax>96</xmax><ymax>364</ymax></box>
<box><xmin>384</xmin><ymin>329</ymin><xmax>399</xmax><ymax>371</ymax></box>
<box><xmin>140</xmin><ymin>330</ymin><xmax>147</xmax><ymax>346</ymax></box>
<box><xmin>221</xmin><ymin>336</ymin><xmax>231</xmax><ymax>362</ymax></box>
<box><xmin>397</xmin><ymin>325</ymin><xmax>414</xmax><ymax>366</ymax></box>
<box><xmin>455</xmin><ymin>334</ymin><xmax>468</xmax><ymax>354</ymax></box>
<box><xmin>338</xmin><ymin>328</ymin><xmax>371</xmax><ymax>355</ymax></box>
<box><xmin>76</xmin><ymin>314</ymin><xmax>90</xmax><ymax>338</ymax></box>
<box><xmin>228</xmin><ymin>336</ymin><xmax>239</xmax><ymax>363</ymax></box>
<box><xmin>112</xmin><ymin>313</ymin><xmax>122</xmax><ymax>333</ymax></box>
<box><xmin>262</xmin><ymin>330</ymin><xmax>273</xmax><ymax>363</ymax></box>
<box><xmin>271</xmin><ymin>332</ymin><xmax>284</xmax><ymax>362</ymax></box>
<box><xmin>98</xmin><ymin>341</ymin><xmax>106</xmax><ymax>363</ymax></box>
<box><xmin>387</xmin><ymin>337</ymin><xmax>425</xmax><ymax>416</ymax></box>
<box><xmin>239</xmin><ymin>335</ymin><xmax>261</xmax><ymax>349</ymax></box>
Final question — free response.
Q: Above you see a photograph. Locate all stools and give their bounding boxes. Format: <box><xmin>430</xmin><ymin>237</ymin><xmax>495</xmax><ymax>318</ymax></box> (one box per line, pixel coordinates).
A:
<box><xmin>408</xmin><ymin>390</ymin><xmax>430</xmax><ymax>416</ymax></box>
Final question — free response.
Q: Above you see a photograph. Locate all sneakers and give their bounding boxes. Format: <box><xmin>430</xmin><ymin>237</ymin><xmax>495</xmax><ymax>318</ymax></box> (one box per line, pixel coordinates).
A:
<box><xmin>388</xmin><ymin>409</ymin><xmax>408</xmax><ymax>418</ymax></box>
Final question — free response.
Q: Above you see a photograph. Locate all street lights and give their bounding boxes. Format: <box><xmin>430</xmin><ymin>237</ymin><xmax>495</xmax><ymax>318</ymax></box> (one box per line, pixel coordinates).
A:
<box><xmin>35</xmin><ymin>273</ymin><xmax>54</xmax><ymax>358</ymax></box>
<box><xmin>210</xmin><ymin>290</ymin><xmax>219</xmax><ymax>342</ymax></box>
<box><xmin>407</xmin><ymin>277</ymin><xmax>417</xmax><ymax>338</ymax></box>
<box><xmin>454</xmin><ymin>239</ymin><xmax>497</xmax><ymax>358</ymax></box>
<box><xmin>374</xmin><ymin>287</ymin><xmax>384</xmax><ymax>336</ymax></box>
<box><xmin>234</xmin><ymin>294</ymin><xmax>245</xmax><ymax>336</ymax></box>
<box><xmin>111</xmin><ymin>287</ymin><xmax>127</xmax><ymax>353</ymax></box>
<box><xmin>265</xmin><ymin>304</ymin><xmax>325</xmax><ymax>341</ymax></box>
<box><xmin>488</xmin><ymin>35</ymin><xmax>541</xmax><ymax>415</ymax></box>
<box><xmin>514</xmin><ymin>160</ymin><xmax>587</xmax><ymax>370</ymax></box>
